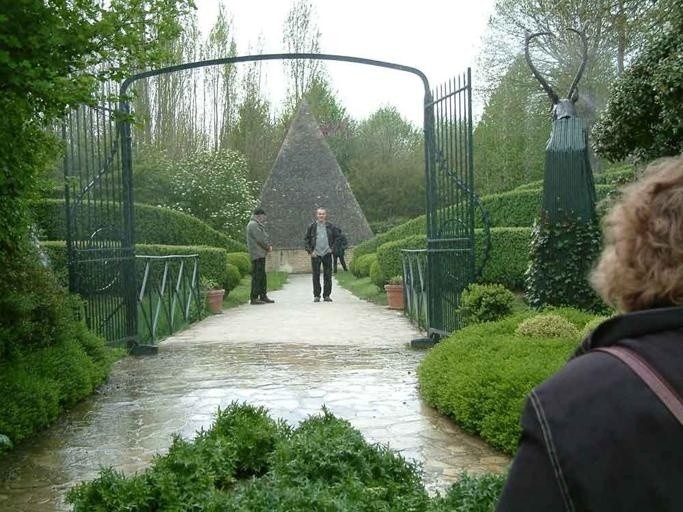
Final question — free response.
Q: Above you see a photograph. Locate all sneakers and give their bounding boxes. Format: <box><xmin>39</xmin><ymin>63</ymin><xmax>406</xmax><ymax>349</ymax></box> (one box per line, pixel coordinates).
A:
<box><xmin>312</xmin><ymin>295</ymin><xmax>333</xmax><ymax>302</ymax></box>
<box><xmin>249</xmin><ymin>296</ymin><xmax>275</xmax><ymax>305</ymax></box>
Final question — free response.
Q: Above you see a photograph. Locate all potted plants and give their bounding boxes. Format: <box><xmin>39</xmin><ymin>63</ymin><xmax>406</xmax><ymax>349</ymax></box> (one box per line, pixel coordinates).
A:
<box><xmin>383</xmin><ymin>275</ymin><xmax>404</xmax><ymax>309</ymax></box>
<box><xmin>198</xmin><ymin>274</ymin><xmax>226</xmax><ymax>314</ymax></box>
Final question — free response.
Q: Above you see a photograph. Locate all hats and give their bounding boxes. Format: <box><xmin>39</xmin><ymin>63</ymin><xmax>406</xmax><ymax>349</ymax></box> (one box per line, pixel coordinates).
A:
<box><xmin>252</xmin><ymin>207</ymin><xmax>265</xmax><ymax>215</ymax></box>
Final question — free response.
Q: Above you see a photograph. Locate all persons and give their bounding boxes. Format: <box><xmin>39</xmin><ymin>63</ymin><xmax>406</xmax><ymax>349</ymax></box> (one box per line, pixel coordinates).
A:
<box><xmin>495</xmin><ymin>154</ymin><xmax>683</xmax><ymax>511</ymax></box>
<box><xmin>332</xmin><ymin>229</ymin><xmax>348</xmax><ymax>273</ymax></box>
<box><xmin>303</xmin><ymin>206</ymin><xmax>339</xmax><ymax>302</ymax></box>
<box><xmin>245</xmin><ymin>208</ymin><xmax>274</xmax><ymax>304</ymax></box>
